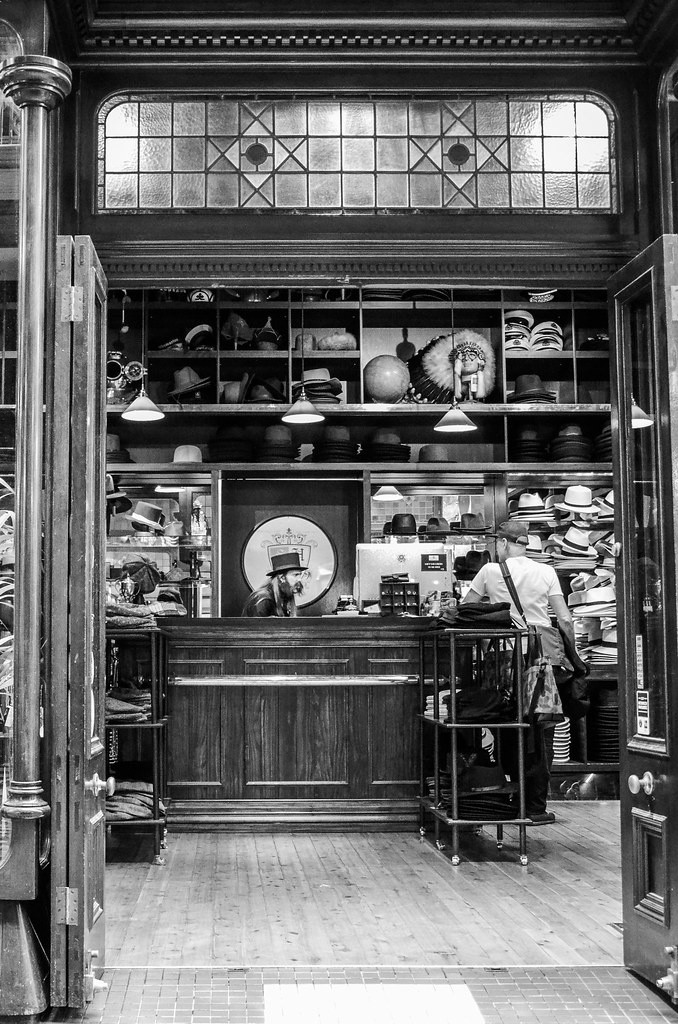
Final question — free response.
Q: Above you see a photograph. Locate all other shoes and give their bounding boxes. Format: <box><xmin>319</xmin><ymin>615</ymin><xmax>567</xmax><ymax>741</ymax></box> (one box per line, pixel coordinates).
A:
<box><xmin>525</xmin><ymin>812</ymin><xmax>555</xmax><ymax>825</ymax></box>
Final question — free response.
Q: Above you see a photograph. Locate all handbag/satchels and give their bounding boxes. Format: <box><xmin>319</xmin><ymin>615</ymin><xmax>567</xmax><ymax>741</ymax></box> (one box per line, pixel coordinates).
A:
<box><xmin>527</xmin><ymin>625</ymin><xmax>574</xmax><ymax>684</ymax></box>
<box><xmin>522</xmin><ymin>626</ymin><xmax>565</xmax><ymax>732</ymax></box>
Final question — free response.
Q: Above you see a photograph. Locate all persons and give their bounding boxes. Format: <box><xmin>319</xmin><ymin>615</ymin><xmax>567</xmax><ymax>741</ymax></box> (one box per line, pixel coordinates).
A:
<box><xmin>241</xmin><ymin>552</ymin><xmax>309</xmax><ymax>618</ymax></box>
<box><xmin>459</xmin><ymin>521</ymin><xmax>591</xmax><ymax>824</ymax></box>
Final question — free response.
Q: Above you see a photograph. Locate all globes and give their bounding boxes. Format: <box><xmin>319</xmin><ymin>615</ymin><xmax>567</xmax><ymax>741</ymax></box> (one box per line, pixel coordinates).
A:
<box><xmin>363</xmin><ymin>355</ymin><xmax>410</xmax><ymax>403</ymax></box>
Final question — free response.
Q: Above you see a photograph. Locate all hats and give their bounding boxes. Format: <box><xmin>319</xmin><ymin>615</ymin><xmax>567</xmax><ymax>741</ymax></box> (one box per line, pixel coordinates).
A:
<box><xmin>594</xmin><ymin>689</ymin><xmax>620</xmax><ymax>762</ymax></box>
<box><xmin>172</xmin><ymin>445</ymin><xmax>204</xmax><ymax>464</ymax></box>
<box><xmin>451</xmin><ymin>550</ymin><xmax>493</xmax><ymax>582</ymax></box>
<box><xmin>526</xmin><ymin>525</ymin><xmax>617</xmax><ymax>569</ymax></box>
<box><xmin>424</xmin><ymin>686</ymin><xmax>516</xmax><ymax>724</ymax></box>
<box><xmin>426</xmin><ymin>746</ymin><xmax>520</xmax><ymax>821</ymax></box>
<box><xmin>125</xmin><ymin>501</ymin><xmax>164</xmax><ymax>531</ymax></box>
<box><xmin>153</xmin><ymin>316</ymin><xmax>356</xmax><ymax>404</ymax></box>
<box><xmin>381</xmin><ymin>512</ymin><xmax>493</xmax><ymax>535</ymax></box>
<box><xmin>510</xmin><ymin>484</ymin><xmax>615</xmax><ymax>527</ymax></box>
<box><xmin>265</xmin><ymin>553</ymin><xmax>308</xmax><ymax>576</ymax></box>
<box><xmin>260</xmin><ymin>425</ymin><xmax>412</xmax><ymax>464</ymax></box>
<box><xmin>547</xmin><ymin>569</ymin><xmax>619</xmax><ymax>665</ymax></box>
<box><xmin>157</xmin><ymin>589</ymin><xmax>183</xmax><ymax>604</ymax></box>
<box><xmin>123</xmin><ymin>554</ymin><xmax>158</xmax><ymax>593</ymax></box>
<box><xmin>515</xmin><ymin>426</ymin><xmax>613</xmax><ymax>461</ymax></box>
<box><xmin>415</xmin><ymin>445</ymin><xmax>457</xmax><ymax>463</ymax></box>
<box><xmin>493</xmin><ymin>521</ymin><xmax>529</xmax><ymax>546</ymax></box>
<box><xmin>504</xmin><ymin>310</ymin><xmax>566</xmax><ymax>403</ymax></box>
<box><xmin>93</xmin><ymin>434</ymin><xmax>135</xmax><ymax>463</ymax></box>
<box><xmin>104</xmin><ymin>474</ymin><xmax>127</xmax><ymax>498</ymax></box>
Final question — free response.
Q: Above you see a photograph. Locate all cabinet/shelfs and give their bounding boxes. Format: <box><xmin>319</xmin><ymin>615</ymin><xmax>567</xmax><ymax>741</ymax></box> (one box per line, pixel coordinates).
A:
<box><xmin>415</xmin><ymin>626</ymin><xmax>530</xmax><ymax>866</ymax></box>
<box><xmin>98</xmin><ymin>297</ymin><xmax>618</xmax><ymax>470</ymax></box>
<box><xmin>104</xmin><ymin>626</ymin><xmax>172</xmax><ymax>866</ymax></box>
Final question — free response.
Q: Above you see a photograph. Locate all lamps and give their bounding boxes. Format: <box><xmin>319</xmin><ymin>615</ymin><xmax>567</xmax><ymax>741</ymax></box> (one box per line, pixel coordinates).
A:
<box><xmin>119</xmin><ymin>288</ymin><xmax>165</xmax><ymax>422</ymax></box>
<box><xmin>283</xmin><ymin>279</ymin><xmax>324</xmax><ymax>427</ymax></box>
<box><xmin>624</xmin><ymin>393</ymin><xmax>654</xmax><ymax>429</ymax></box>
<box><xmin>372</xmin><ymin>484</ymin><xmax>403</xmax><ymax>502</ymax></box>
<box><xmin>434</xmin><ymin>291</ymin><xmax>478</xmax><ymax>432</ymax></box>
